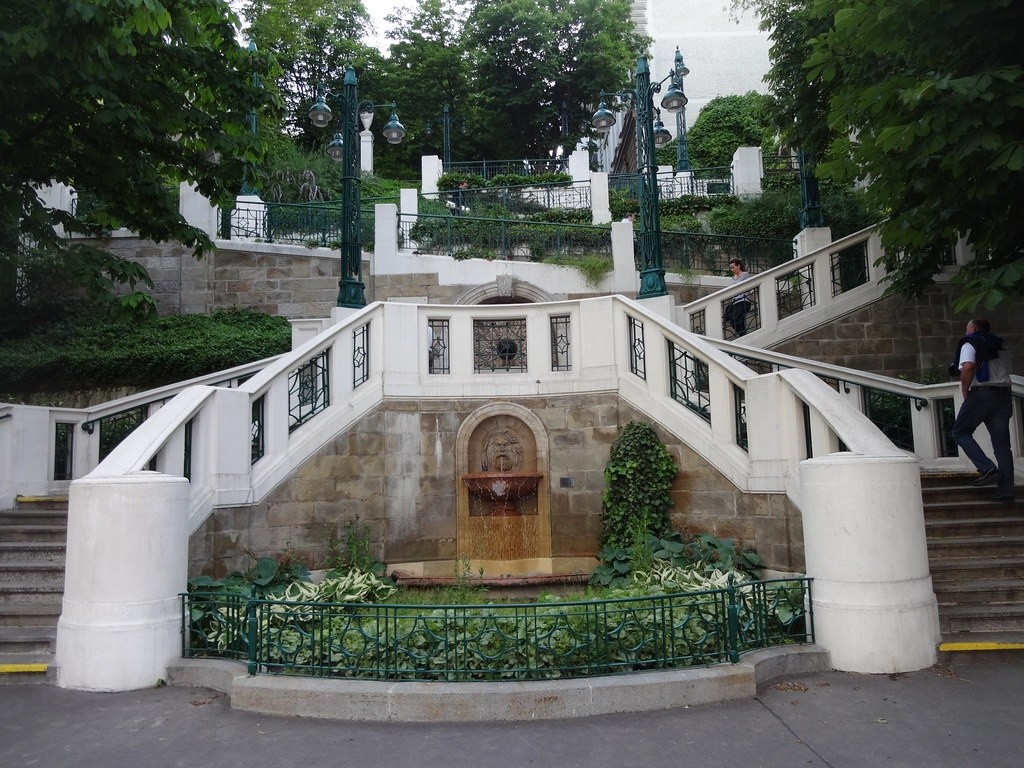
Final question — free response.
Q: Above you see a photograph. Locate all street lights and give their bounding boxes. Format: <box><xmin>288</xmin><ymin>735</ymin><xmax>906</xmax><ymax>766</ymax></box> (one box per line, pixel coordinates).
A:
<box><xmin>593</xmin><ymin>44</ymin><xmax>689</xmax><ymax>300</ymax></box>
<box><xmin>307</xmin><ymin>56</ymin><xmax>407</xmax><ymax>309</ymax></box>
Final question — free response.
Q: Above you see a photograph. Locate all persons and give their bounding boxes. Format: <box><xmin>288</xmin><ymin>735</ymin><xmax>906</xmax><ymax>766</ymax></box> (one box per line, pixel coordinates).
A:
<box><xmin>726</xmin><ymin>259</ymin><xmax>750</xmax><ymax>336</ymax></box>
<box><xmin>952</xmin><ymin>319</ymin><xmax>1015</xmax><ymax>504</ymax></box>
<box><xmin>451</xmin><ymin>181</ymin><xmax>467</xmax><ymax>216</ymax></box>
<box><xmin>622</xmin><ymin>214</ymin><xmax>638</xmax><ymax>259</ymax></box>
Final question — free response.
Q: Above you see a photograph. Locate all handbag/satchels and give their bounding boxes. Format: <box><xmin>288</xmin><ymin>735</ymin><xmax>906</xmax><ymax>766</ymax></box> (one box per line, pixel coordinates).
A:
<box><xmin>725</xmin><ymin>305</ymin><xmax>733</xmax><ymax>322</ymax></box>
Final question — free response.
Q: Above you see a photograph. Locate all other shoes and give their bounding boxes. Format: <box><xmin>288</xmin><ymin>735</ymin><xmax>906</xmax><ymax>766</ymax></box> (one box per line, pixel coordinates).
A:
<box><xmin>972</xmin><ymin>467</ymin><xmax>999</xmax><ymax>486</ymax></box>
<box><xmin>988</xmin><ymin>493</ymin><xmax>1015</xmax><ymax>502</ymax></box>
